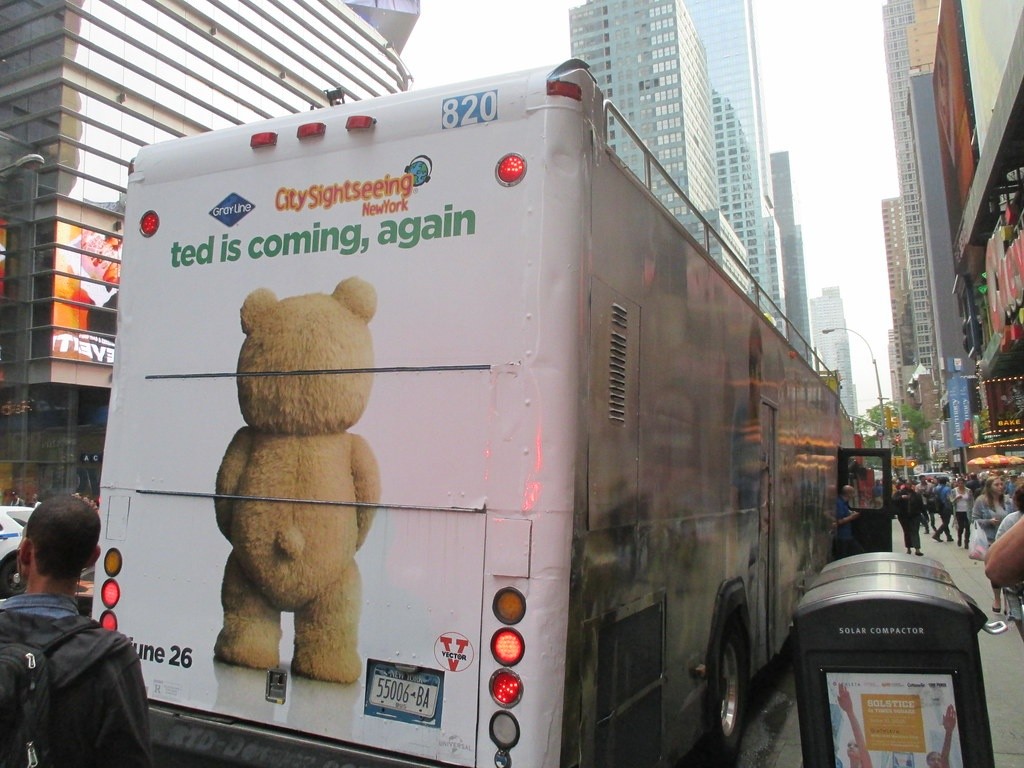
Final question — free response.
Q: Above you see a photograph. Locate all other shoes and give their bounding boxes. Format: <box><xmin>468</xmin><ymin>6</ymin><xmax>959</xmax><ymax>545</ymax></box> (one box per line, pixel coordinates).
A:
<box><xmin>924</xmin><ymin>530</ymin><xmax>929</xmax><ymax>534</ymax></box>
<box><xmin>965</xmin><ymin>545</ymin><xmax>969</xmax><ymax>549</ymax></box>
<box><xmin>932</xmin><ymin>536</ymin><xmax>943</xmax><ymax>542</ymax></box>
<box><xmin>948</xmin><ymin>538</ymin><xmax>954</xmax><ymax>541</ymax></box>
<box><xmin>957</xmin><ymin>539</ymin><xmax>961</xmax><ymax>547</ymax></box>
<box><xmin>992</xmin><ymin>603</ymin><xmax>1002</xmax><ymax>613</ymax></box>
<box><xmin>915</xmin><ymin>551</ymin><xmax>923</xmax><ymax>556</ymax></box>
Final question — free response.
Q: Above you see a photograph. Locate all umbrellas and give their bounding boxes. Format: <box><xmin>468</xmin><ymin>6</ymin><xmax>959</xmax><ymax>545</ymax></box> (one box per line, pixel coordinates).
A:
<box><xmin>968</xmin><ymin>454</ymin><xmax>1024</xmax><ymax>466</ymax></box>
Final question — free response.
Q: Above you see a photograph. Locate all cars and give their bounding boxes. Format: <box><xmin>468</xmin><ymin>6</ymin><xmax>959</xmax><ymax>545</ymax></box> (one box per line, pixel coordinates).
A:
<box><xmin>0</xmin><ymin>504</ymin><xmax>38</xmax><ymax>598</ymax></box>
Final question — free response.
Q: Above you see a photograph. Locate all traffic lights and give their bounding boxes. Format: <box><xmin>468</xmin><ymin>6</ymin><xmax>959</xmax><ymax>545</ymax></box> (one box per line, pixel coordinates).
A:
<box><xmin>906</xmin><ymin>460</ymin><xmax>918</xmax><ymax>467</ymax></box>
<box><xmin>891</xmin><ymin>416</ymin><xmax>899</xmax><ymax>431</ymax></box>
<box><xmin>882</xmin><ymin>404</ymin><xmax>892</xmax><ymax>430</ymax></box>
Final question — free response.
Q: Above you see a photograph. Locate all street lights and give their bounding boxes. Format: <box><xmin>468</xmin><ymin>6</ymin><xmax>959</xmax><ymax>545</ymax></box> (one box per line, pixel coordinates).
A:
<box><xmin>822</xmin><ymin>327</ymin><xmax>890</xmax><ymax>440</ymax></box>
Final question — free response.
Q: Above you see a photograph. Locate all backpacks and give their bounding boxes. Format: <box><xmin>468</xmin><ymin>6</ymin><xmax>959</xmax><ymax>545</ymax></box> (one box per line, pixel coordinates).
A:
<box><xmin>0</xmin><ymin>621</ymin><xmax>102</xmax><ymax>768</ymax></box>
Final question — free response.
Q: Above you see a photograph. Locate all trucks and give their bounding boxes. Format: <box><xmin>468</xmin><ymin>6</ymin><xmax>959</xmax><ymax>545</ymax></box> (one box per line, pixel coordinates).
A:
<box><xmin>87</xmin><ymin>61</ymin><xmax>892</xmax><ymax>768</ymax></box>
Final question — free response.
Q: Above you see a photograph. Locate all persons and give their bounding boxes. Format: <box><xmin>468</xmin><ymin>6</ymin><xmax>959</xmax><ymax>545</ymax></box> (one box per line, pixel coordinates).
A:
<box><xmin>0</xmin><ymin>492</ymin><xmax>156</xmax><ymax>768</ymax></box>
<box><xmin>8</xmin><ymin>489</ymin><xmax>99</xmax><ymax>513</ymax></box>
<box><xmin>879</xmin><ymin>466</ymin><xmax>1024</xmax><ymax>645</ymax></box>
<box><xmin>924</xmin><ymin>704</ymin><xmax>957</xmax><ymax>768</ymax></box>
<box><xmin>729</xmin><ymin>316</ymin><xmax>770</xmax><ymax>602</ymax></box>
<box><xmin>836</xmin><ymin>485</ymin><xmax>860</xmax><ymax>559</ymax></box>
<box><xmin>835</xmin><ymin>680</ymin><xmax>875</xmax><ymax>768</ymax></box>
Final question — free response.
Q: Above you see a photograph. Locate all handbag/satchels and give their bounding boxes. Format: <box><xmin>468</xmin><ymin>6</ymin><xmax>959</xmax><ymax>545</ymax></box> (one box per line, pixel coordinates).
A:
<box><xmin>968</xmin><ymin>521</ymin><xmax>989</xmax><ymax>561</ymax></box>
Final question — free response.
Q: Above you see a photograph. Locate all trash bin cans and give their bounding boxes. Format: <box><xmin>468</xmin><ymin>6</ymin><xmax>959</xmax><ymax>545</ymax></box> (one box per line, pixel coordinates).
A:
<box><xmin>775</xmin><ymin>552</ymin><xmax>1006</xmax><ymax>768</ymax></box>
